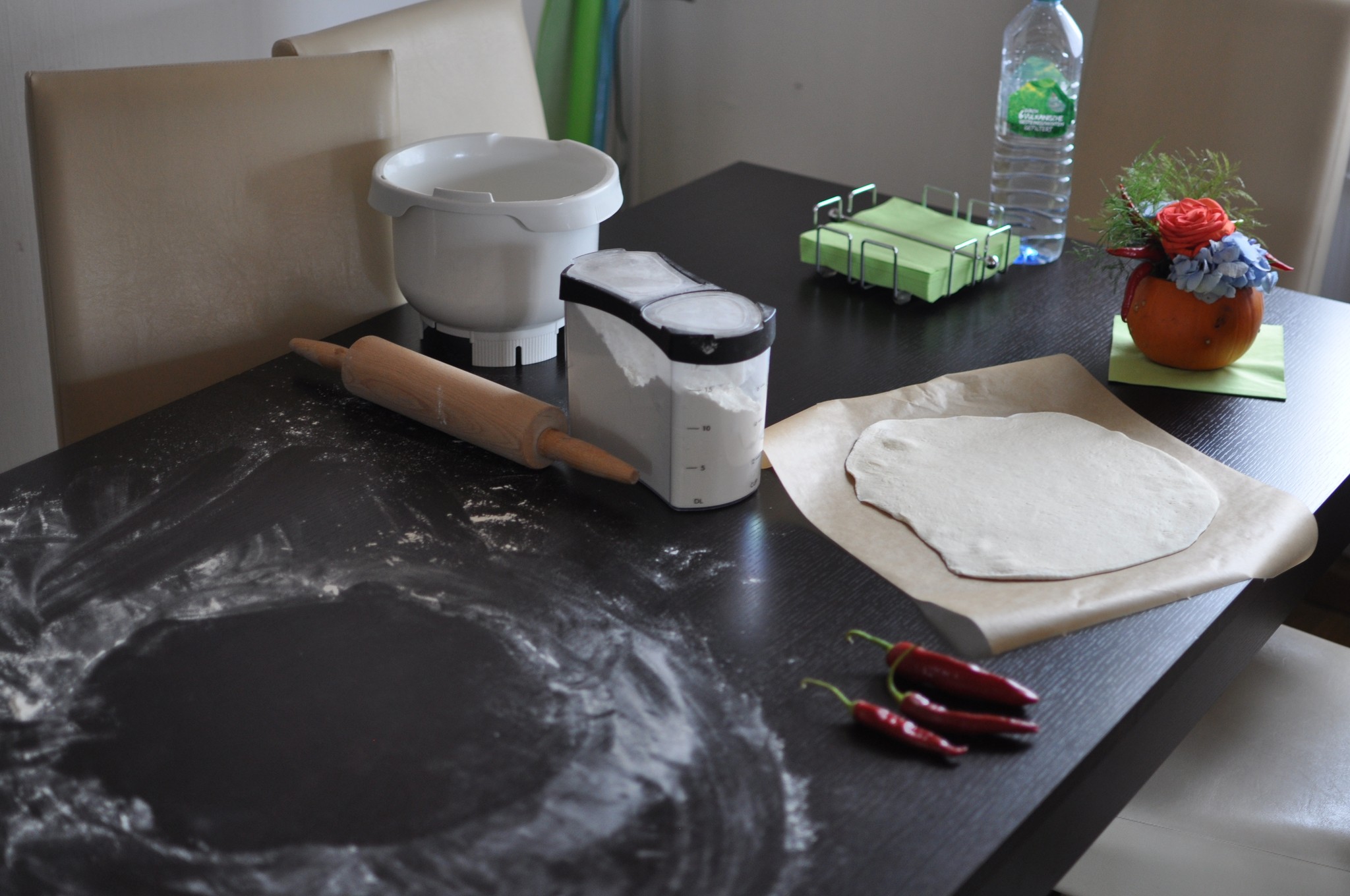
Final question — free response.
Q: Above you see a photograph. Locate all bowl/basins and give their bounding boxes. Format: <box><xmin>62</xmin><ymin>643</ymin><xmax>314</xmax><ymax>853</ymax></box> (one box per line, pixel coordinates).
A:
<box><xmin>367</xmin><ymin>132</ymin><xmax>623</xmax><ymax>367</ymax></box>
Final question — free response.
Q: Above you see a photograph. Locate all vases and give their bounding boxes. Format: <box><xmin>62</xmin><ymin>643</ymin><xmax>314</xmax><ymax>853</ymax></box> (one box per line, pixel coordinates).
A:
<box><xmin>1124</xmin><ymin>275</ymin><xmax>1263</xmax><ymax>368</ymax></box>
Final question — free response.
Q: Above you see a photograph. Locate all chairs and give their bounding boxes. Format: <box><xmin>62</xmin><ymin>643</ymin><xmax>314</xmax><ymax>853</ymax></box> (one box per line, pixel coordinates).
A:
<box><xmin>272</xmin><ymin>0</ymin><xmax>559</xmax><ymax>154</ymax></box>
<box><xmin>1029</xmin><ymin>609</ymin><xmax>1350</xmax><ymax>896</ymax></box>
<box><xmin>27</xmin><ymin>52</ymin><xmax>403</xmax><ymax>456</ymax></box>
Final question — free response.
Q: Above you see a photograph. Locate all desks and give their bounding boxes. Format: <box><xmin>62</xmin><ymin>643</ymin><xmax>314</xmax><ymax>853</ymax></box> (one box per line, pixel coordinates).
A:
<box><xmin>1</xmin><ymin>159</ymin><xmax>1350</xmax><ymax>896</ymax></box>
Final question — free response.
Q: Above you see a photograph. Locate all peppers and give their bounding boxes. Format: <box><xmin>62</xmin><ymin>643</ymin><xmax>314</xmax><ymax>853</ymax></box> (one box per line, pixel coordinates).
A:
<box><xmin>799</xmin><ymin>618</ymin><xmax>1039</xmax><ymax>766</ymax></box>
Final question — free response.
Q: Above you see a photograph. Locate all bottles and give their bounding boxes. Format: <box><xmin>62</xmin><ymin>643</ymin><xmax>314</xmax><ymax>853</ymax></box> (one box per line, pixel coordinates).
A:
<box><xmin>987</xmin><ymin>0</ymin><xmax>1085</xmax><ymax>266</ymax></box>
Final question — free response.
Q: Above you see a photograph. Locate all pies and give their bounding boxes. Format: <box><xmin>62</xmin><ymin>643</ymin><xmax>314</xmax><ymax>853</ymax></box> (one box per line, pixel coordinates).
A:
<box><xmin>842</xmin><ymin>395</ymin><xmax>1223</xmax><ymax>583</ymax></box>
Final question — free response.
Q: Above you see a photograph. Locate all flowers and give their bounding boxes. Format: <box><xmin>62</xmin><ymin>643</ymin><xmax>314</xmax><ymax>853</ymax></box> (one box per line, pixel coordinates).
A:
<box><xmin>1071</xmin><ymin>135</ymin><xmax>1295</xmax><ymax>327</ymax></box>
